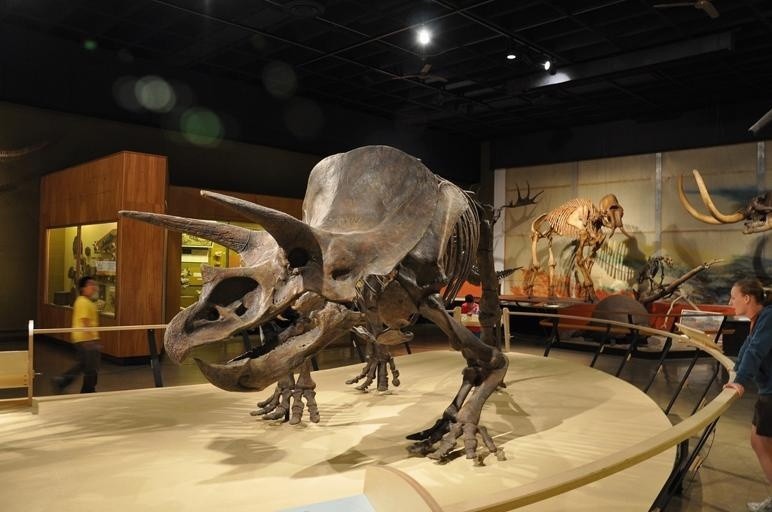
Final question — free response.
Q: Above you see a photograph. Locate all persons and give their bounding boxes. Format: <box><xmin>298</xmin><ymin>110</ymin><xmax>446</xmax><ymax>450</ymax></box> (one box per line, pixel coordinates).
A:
<box><xmin>51</xmin><ymin>276</ymin><xmax>102</xmax><ymax>394</ymax></box>
<box><xmin>722</xmin><ymin>277</ymin><xmax>772</xmax><ymax>512</ymax></box>
<box><xmin>461</xmin><ymin>294</ymin><xmax>482</xmax><ymax>339</ymax></box>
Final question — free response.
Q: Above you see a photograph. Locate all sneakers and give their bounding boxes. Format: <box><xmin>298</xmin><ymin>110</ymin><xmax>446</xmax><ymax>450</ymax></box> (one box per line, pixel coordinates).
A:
<box><xmin>747</xmin><ymin>498</ymin><xmax>772</xmax><ymax>512</ymax></box>
